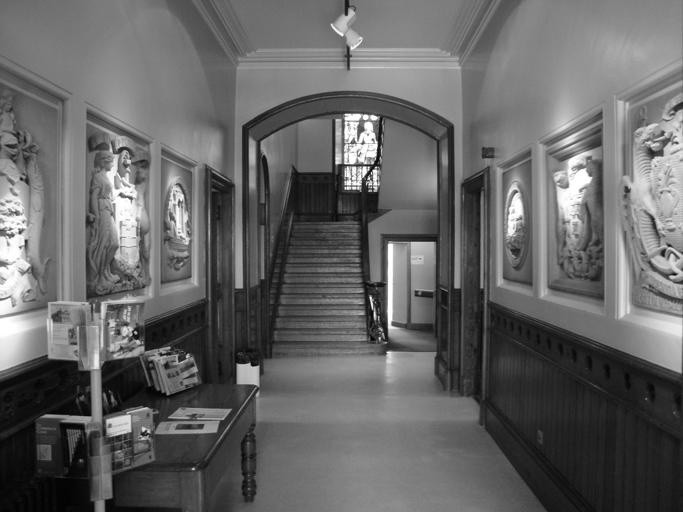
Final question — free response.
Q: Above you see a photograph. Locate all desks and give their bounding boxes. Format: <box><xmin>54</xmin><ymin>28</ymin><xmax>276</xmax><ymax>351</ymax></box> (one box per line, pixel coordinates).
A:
<box><xmin>64</xmin><ymin>383</ymin><xmax>260</xmax><ymax>512</ymax></box>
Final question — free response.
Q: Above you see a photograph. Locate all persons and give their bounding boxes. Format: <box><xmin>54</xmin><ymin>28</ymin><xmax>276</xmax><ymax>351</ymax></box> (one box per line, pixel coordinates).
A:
<box><xmin>0</xmin><ymin>96</ymin><xmax>16</xmax><ymax>132</ymax></box>
<box><xmin>86</xmin><ymin>148</ymin><xmax>120</xmax><ymax>297</ymax></box>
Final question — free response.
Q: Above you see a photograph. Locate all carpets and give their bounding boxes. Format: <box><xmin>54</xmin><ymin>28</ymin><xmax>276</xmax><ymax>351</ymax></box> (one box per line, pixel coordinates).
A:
<box><xmin>388</xmin><ymin>327</ymin><xmax>444</xmax><ymax>354</ymax></box>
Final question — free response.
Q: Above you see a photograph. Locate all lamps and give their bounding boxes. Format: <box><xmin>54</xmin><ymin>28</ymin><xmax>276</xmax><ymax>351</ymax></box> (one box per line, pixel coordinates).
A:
<box><xmin>329</xmin><ymin>0</ymin><xmax>364</xmax><ymax>53</ymax></box>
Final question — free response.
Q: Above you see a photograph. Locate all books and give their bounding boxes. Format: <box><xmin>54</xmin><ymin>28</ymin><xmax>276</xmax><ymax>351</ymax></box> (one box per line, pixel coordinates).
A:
<box><xmin>33</xmin><ymin>298</ymin><xmax>155</xmax><ymax>480</ymax></box>
<box><xmin>139</xmin><ymin>346</ymin><xmax>203</xmax><ymax>396</ymax></box>
